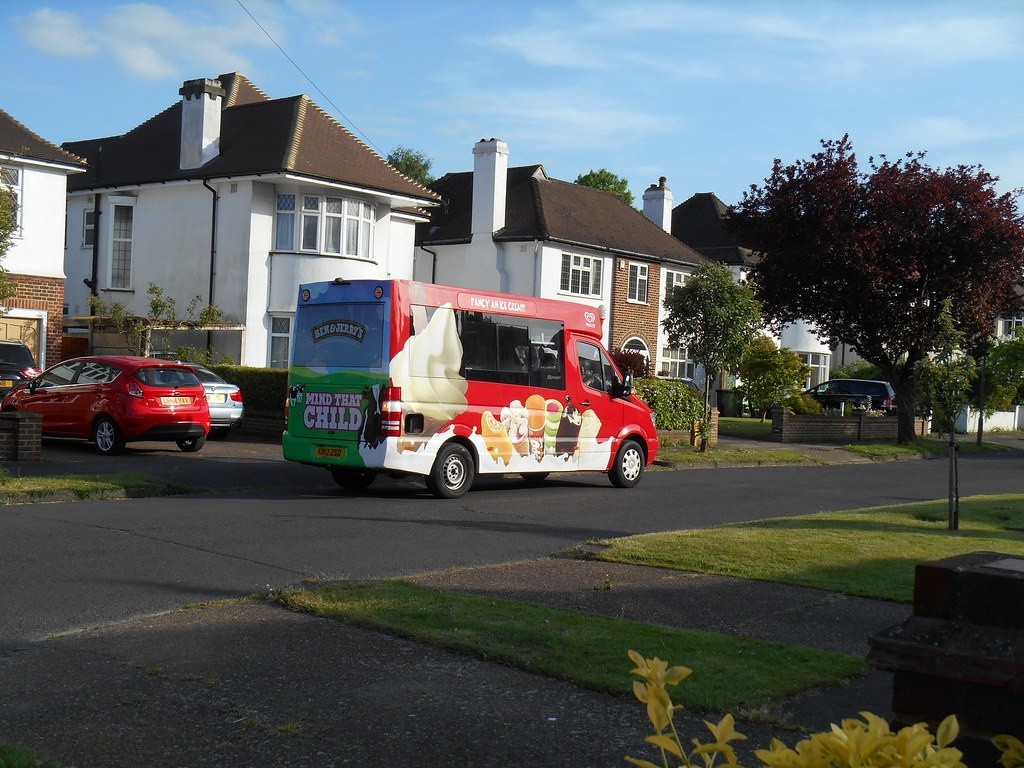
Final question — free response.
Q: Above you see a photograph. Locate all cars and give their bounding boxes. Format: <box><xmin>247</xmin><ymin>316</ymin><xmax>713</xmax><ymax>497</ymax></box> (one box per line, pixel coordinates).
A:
<box><xmin>181</xmin><ymin>363</ymin><xmax>245</xmax><ymax>441</ymax></box>
<box><xmin>0</xmin><ymin>354</ymin><xmax>212</xmax><ymax>456</ymax></box>
<box><xmin>800</xmin><ymin>378</ymin><xmax>896</xmax><ymax>412</ymax></box>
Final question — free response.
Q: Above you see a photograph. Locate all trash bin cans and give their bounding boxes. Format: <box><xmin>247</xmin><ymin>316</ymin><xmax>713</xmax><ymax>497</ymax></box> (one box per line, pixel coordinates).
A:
<box><xmin>715</xmin><ymin>389</ymin><xmax>744</xmax><ymax>418</ymax></box>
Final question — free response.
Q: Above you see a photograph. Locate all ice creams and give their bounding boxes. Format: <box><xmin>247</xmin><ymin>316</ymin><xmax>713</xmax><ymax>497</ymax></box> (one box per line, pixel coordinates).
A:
<box><xmin>499</xmin><ymin>400</ymin><xmax>530</xmax><ymax>456</ymax></box>
<box><xmin>389</xmin><ymin>303</ymin><xmax>468</xmax><ymax>453</ymax></box>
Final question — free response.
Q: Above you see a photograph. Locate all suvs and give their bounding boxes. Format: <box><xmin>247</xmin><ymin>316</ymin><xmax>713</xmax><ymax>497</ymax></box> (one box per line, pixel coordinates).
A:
<box><xmin>0</xmin><ymin>337</ymin><xmax>42</xmax><ymax>402</ymax></box>
<box><xmin>656</xmin><ymin>376</ymin><xmax>711</xmax><ymax>411</ymax></box>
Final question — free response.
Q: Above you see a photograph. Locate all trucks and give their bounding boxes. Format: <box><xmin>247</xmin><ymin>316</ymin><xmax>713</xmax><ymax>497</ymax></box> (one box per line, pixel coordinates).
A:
<box><xmin>282</xmin><ymin>276</ymin><xmax>660</xmax><ymax>499</ymax></box>
